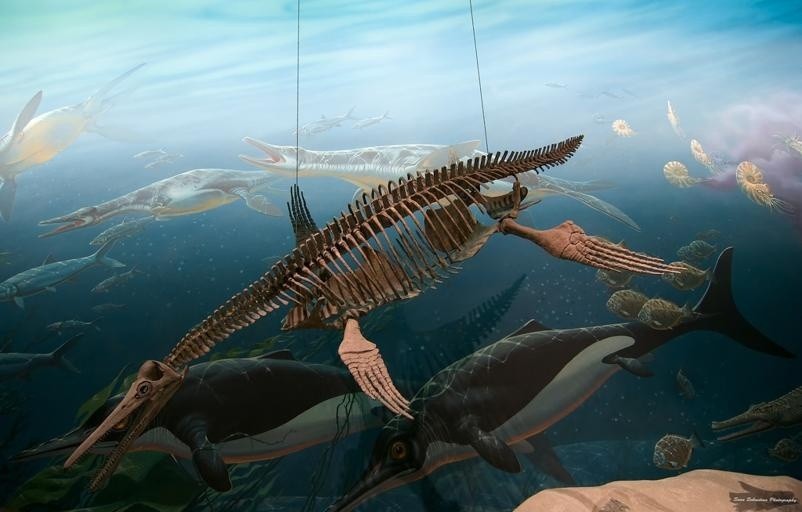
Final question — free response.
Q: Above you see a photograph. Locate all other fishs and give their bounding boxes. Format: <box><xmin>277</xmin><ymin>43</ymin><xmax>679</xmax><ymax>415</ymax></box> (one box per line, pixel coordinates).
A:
<box><xmin>0</xmin><ymin>63</ymin><xmax>802</xmax><ymax>511</ymax></box>
